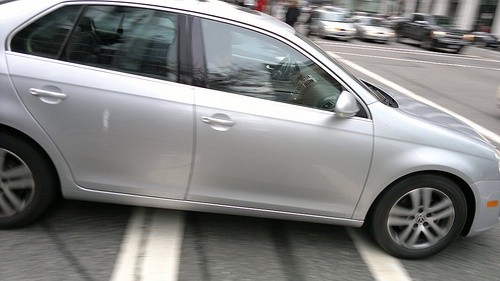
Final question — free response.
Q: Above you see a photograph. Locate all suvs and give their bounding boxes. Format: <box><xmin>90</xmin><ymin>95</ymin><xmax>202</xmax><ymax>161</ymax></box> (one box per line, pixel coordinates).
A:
<box><xmin>398</xmin><ymin>12</ymin><xmax>476</xmax><ymax>53</ymax></box>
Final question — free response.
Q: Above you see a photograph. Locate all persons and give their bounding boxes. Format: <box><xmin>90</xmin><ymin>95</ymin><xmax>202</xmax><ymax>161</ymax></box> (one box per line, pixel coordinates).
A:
<box><xmin>285</xmin><ymin>1</ymin><xmax>301</xmax><ymax>28</ymax></box>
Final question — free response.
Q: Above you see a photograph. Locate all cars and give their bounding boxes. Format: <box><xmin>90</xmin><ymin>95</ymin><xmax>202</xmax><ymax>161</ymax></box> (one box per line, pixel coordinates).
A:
<box><xmin>474</xmin><ymin>31</ymin><xmax>500</xmax><ymax>48</ymax></box>
<box><xmin>0</xmin><ymin>0</ymin><xmax>500</xmax><ymax>259</ymax></box>
<box><xmin>310</xmin><ymin>10</ymin><xmax>357</xmax><ymax>40</ymax></box>
<box><xmin>352</xmin><ymin>15</ymin><xmax>397</xmax><ymax>44</ymax></box>
